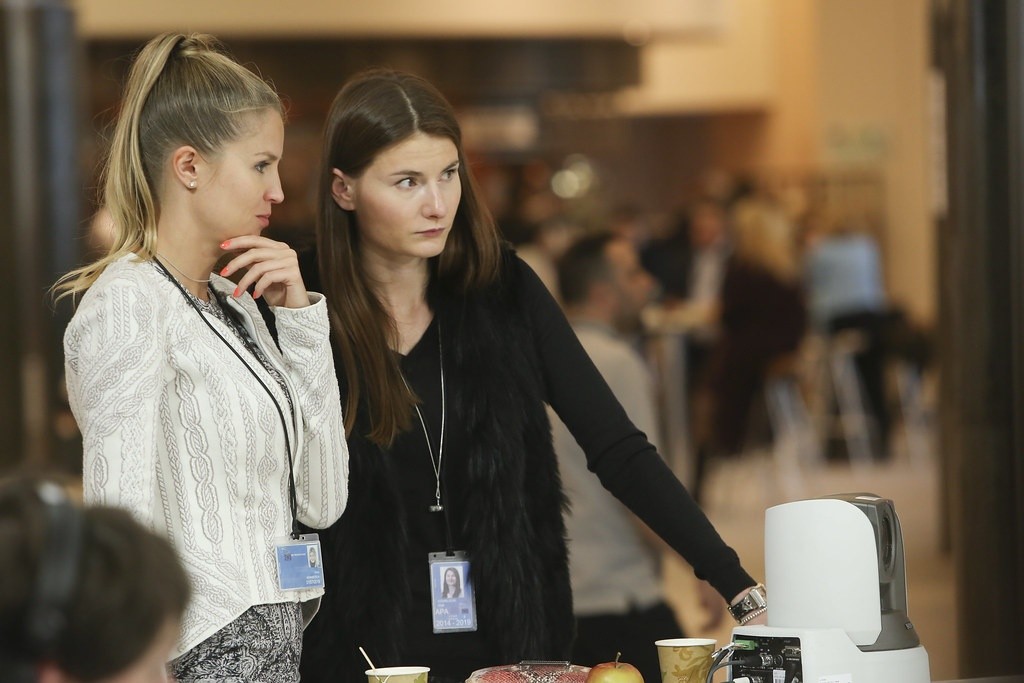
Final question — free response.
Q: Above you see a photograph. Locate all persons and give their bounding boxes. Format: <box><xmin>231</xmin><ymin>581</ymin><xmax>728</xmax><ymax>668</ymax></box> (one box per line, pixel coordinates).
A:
<box><xmin>41</xmin><ymin>25</ymin><xmax>350</xmax><ymax>683</ymax></box>
<box><xmin>309</xmin><ymin>546</ymin><xmax>319</xmax><ymax>568</ymax></box>
<box><xmin>0</xmin><ymin>479</ymin><xmax>191</xmax><ymax>683</ymax></box>
<box><xmin>634</xmin><ymin>166</ymin><xmax>932</xmax><ymax>505</ymax></box>
<box><xmin>543</xmin><ymin>227</ymin><xmax>693</xmax><ymax>683</ymax></box>
<box><xmin>496</xmin><ymin>154</ymin><xmax>569</xmax><ymax>249</ymax></box>
<box><xmin>245</xmin><ymin>65</ymin><xmax>768</xmax><ymax>683</ymax></box>
<box><xmin>440</xmin><ymin>567</ymin><xmax>463</xmax><ymax>597</ymax></box>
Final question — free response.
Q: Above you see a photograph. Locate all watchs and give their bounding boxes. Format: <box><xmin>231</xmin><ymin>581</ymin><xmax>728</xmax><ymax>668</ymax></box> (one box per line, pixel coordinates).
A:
<box><xmin>727</xmin><ymin>582</ymin><xmax>767</xmax><ymax>623</ymax></box>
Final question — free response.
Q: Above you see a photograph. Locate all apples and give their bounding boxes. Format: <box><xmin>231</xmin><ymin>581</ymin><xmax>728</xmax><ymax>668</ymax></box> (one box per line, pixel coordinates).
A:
<box><xmin>585</xmin><ymin>652</ymin><xmax>645</xmax><ymax>682</ymax></box>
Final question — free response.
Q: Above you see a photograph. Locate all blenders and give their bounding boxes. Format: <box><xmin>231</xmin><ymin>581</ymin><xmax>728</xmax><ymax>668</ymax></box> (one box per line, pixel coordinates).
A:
<box><xmin>728</xmin><ymin>492</ymin><xmax>930</xmax><ymax>683</ymax></box>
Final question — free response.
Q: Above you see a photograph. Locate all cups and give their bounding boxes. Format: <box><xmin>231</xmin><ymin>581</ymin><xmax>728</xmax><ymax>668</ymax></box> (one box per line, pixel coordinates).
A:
<box><xmin>365</xmin><ymin>666</ymin><xmax>430</xmax><ymax>683</ymax></box>
<box><xmin>655</xmin><ymin>638</ymin><xmax>717</xmax><ymax>683</ymax></box>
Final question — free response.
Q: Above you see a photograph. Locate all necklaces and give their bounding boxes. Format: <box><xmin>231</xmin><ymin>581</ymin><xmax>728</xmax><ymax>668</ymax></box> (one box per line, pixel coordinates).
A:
<box><xmin>157</xmin><ymin>253</ymin><xmax>213</xmax><ymax>282</ymax></box>
<box><xmin>388</xmin><ymin>311</ymin><xmax>445</xmax><ymax>513</ymax></box>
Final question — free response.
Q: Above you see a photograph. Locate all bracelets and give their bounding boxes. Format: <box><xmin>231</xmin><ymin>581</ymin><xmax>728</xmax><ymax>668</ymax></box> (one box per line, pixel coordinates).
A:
<box><xmin>739</xmin><ymin>608</ymin><xmax>767</xmax><ymax>626</ymax></box>
